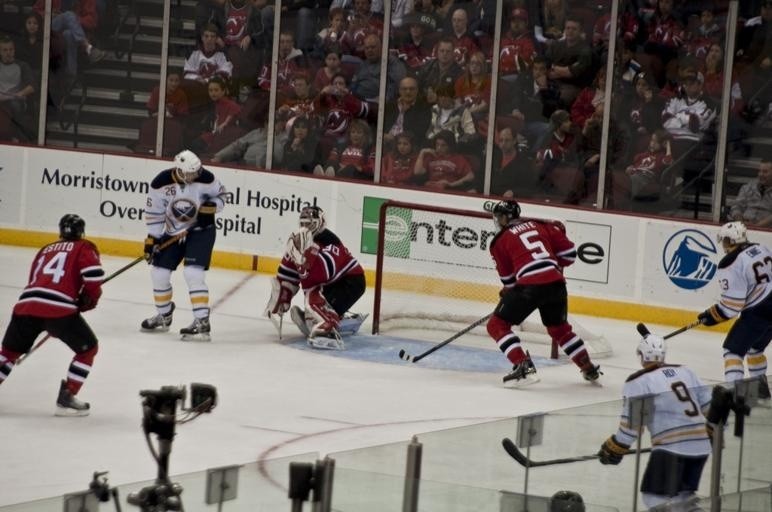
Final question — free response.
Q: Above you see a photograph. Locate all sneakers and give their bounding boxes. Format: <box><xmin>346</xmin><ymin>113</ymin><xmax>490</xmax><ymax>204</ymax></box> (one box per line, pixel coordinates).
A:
<box><xmin>55</xmin><ymin>380</ymin><xmax>91</xmax><ymax>410</ymax></box>
<box><xmin>142</xmin><ymin>304</ymin><xmax>178</xmax><ymax>329</ymax></box>
<box><xmin>291</xmin><ymin>306</ymin><xmax>312</xmax><ymax>337</ymax></box>
<box><xmin>184</xmin><ymin>316</ymin><xmax>210</xmax><ymax>333</ymax></box>
<box><xmin>309</xmin><ymin>331</ymin><xmax>345</xmax><ymax>345</ymax></box>
<box><xmin>753</xmin><ymin>378</ymin><xmax>770</xmax><ymax>397</ymax></box>
<box><xmin>502</xmin><ymin>358</ymin><xmax>537</xmax><ymax>382</ymax></box>
<box><xmin>584</xmin><ymin>366</ymin><xmax>598</xmax><ymax>381</ymax></box>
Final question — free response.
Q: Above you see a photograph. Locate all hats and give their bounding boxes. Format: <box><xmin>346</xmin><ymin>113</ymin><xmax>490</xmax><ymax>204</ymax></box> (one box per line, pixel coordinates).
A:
<box><xmin>636</xmin><ymin>70</ymin><xmax>653</xmax><ymax>81</ymax></box>
<box><xmin>438</xmin><ymin>75</ymin><xmax>455</xmax><ymax>94</ymax></box>
<box><xmin>511</xmin><ymin>8</ymin><xmax>528</xmax><ymax>19</ymax></box>
<box><xmin>551</xmin><ymin>109</ymin><xmax>569</xmax><ymax>123</ymax></box>
<box><xmin>685</xmin><ymin>69</ymin><xmax>705</xmax><ymax>82</ymax></box>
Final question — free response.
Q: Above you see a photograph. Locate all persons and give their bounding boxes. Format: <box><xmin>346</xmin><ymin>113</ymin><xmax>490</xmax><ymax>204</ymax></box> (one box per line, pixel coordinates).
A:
<box><xmin>141</xmin><ymin>148</ymin><xmax>226</xmax><ymax>341</ymax></box>
<box><xmin>264</xmin><ymin>207</ymin><xmax>368</xmax><ymax>350</ymax></box>
<box><xmin>600</xmin><ymin>334</ymin><xmax>724</xmax><ymax>511</ymax></box>
<box><xmin>2</xmin><ymin>215</ymin><xmax>106</xmax><ymax>418</ymax></box>
<box><xmin>484</xmin><ymin>198</ymin><xmax>603</xmax><ymax>387</ymax></box>
<box><xmin>697</xmin><ymin>221</ymin><xmax>772</xmax><ymax>450</ymax></box>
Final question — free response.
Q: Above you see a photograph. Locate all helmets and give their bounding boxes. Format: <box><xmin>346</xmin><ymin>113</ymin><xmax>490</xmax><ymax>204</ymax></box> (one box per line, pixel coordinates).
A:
<box><xmin>299</xmin><ymin>206</ymin><xmax>326</xmax><ymax>234</ymax></box>
<box><xmin>492</xmin><ymin>200</ymin><xmax>521</xmax><ymax>227</ymax></box>
<box><xmin>173</xmin><ymin>150</ymin><xmax>202</xmax><ymax>184</ymax></box>
<box><xmin>716</xmin><ymin>221</ymin><xmax>748</xmax><ymax>244</ymax></box>
<box><xmin>58</xmin><ymin>213</ymin><xmax>85</xmax><ymax>241</ymax></box>
<box><xmin>637</xmin><ymin>335</ymin><xmax>667</xmax><ymax>364</ymax></box>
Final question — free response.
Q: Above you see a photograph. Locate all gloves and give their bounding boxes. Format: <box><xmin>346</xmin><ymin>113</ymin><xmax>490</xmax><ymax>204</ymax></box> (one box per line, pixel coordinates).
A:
<box><xmin>264</xmin><ymin>278</ymin><xmax>296</xmax><ymax>340</ymax></box>
<box><xmin>286</xmin><ymin>228</ymin><xmax>319</xmax><ymax>279</ymax></box>
<box><xmin>196</xmin><ymin>201</ymin><xmax>217</xmax><ymax>227</ymax></box>
<box><xmin>145</xmin><ymin>236</ymin><xmax>156</xmax><ymax>266</ymax></box>
<box><xmin>79</xmin><ymin>288</ymin><xmax>97</xmax><ymax>313</ymax></box>
<box><xmin>598</xmin><ymin>434</ymin><xmax>630</xmax><ymax>466</ymax></box>
<box><xmin>698</xmin><ymin>304</ymin><xmax>730</xmax><ymax>327</ymax></box>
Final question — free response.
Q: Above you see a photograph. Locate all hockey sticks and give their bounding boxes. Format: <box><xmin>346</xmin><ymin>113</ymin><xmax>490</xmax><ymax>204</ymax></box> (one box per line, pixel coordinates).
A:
<box><xmin>266</xmin><ymin>310</ymin><xmax>283</xmax><ymax>339</ymax></box>
<box><xmin>398</xmin><ymin>312</ymin><xmax>492</xmax><ymax>362</ymax></box>
<box><xmin>632</xmin><ymin>320</ymin><xmax>704</xmax><ymax>343</ymax></box>
<box><xmin>498</xmin><ymin>436</ymin><xmax>654</xmax><ymax>468</ymax></box>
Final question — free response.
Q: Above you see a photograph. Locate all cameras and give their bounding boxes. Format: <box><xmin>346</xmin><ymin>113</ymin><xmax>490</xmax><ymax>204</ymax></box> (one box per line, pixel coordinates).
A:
<box><xmin>332</xmin><ymin>87</ymin><xmax>340</xmax><ymax>97</ymax></box>
<box><xmin>140</xmin><ymin>380</ymin><xmax>219</xmax><ymax>441</ymax></box>
